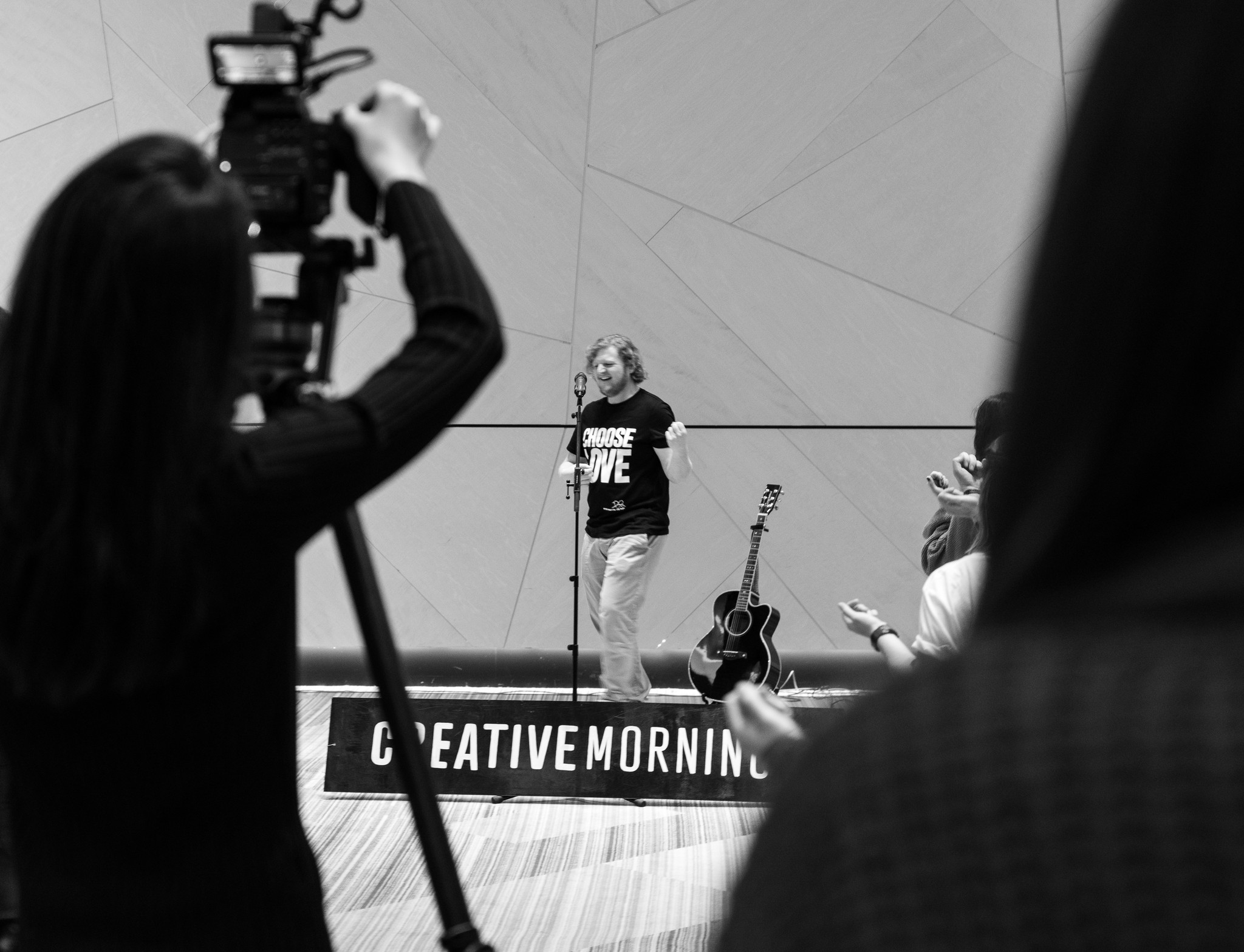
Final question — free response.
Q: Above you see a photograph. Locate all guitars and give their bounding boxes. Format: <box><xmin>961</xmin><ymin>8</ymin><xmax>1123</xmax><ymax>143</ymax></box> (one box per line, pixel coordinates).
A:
<box><xmin>686</xmin><ymin>484</ymin><xmax>788</xmax><ymax>705</ymax></box>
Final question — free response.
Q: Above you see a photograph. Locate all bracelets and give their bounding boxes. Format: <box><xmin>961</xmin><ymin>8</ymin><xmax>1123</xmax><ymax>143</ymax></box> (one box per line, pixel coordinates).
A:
<box><xmin>871</xmin><ymin>625</ymin><xmax>898</xmax><ymax>653</ymax></box>
<box><xmin>963</xmin><ymin>489</ymin><xmax>981</xmax><ymax>495</ymax></box>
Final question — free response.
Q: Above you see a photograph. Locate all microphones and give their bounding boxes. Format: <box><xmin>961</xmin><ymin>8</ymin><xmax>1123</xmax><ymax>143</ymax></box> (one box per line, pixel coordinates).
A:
<box><xmin>574</xmin><ymin>373</ymin><xmax>587</xmax><ymax>396</ymax></box>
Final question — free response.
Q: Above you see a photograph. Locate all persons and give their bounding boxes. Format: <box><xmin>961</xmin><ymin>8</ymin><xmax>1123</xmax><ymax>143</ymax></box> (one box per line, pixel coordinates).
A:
<box><xmin>920</xmin><ymin>394</ymin><xmax>1006</xmax><ymax>575</ymax></box>
<box><xmin>719</xmin><ymin>0</ymin><xmax>1244</xmax><ymax>952</ymax></box>
<box><xmin>0</xmin><ymin>82</ymin><xmax>506</xmax><ymax>952</ymax></box>
<box><xmin>837</xmin><ymin>434</ymin><xmax>1000</xmax><ymax>673</ymax></box>
<box><xmin>558</xmin><ymin>334</ymin><xmax>692</xmax><ymax>701</ymax></box>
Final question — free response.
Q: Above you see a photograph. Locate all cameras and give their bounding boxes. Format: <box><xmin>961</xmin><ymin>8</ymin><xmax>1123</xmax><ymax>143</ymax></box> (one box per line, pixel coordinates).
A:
<box><xmin>206</xmin><ymin>0</ymin><xmax>387</xmax><ymax>392</ymax></box>
